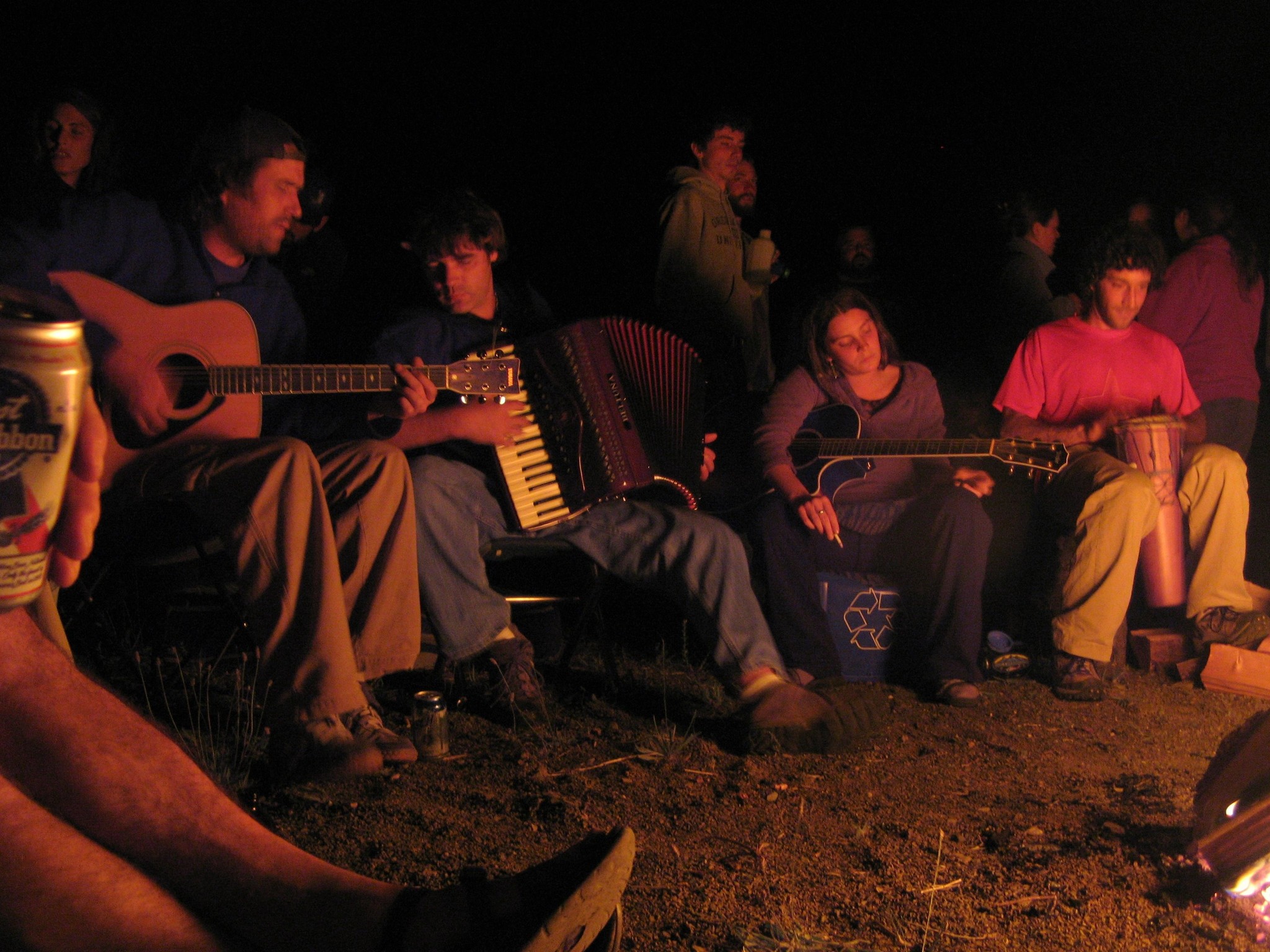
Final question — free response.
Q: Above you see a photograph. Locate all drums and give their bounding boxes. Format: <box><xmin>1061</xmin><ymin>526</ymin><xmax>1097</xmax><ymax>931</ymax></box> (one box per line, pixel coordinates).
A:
<box><xmin>1111</xmin><ymin>413</ymin><xmax>1189</xmax><ymax>611</ymax></box>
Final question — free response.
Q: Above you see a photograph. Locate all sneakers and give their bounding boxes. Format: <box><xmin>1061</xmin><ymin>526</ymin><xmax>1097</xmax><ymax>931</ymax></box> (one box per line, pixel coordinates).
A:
<box><xmin>338</xmin><ymin>708</ymin><xmax>418</xmax><ymax>763</ymax></box>
<box><xmin>1049</xmin><ymin>651</ymin><xmax>1105</xmax><ymax>700</ymax></box>
<box><xmin>1193</xmin><ymin>606</ymin><xmax>1270</xmax><ymax>653</ymax></box>
<box><xmin>493</xmin><ymin>640</ymin><xmax>547</xmax><ymax>720</ymax></box>
<box><xmin>299</xmin><ymin>715</ymin><xmax>383</xmax><ymax>777</ymax></box>
<box><xmin>742</xmin><ymin>683</ymin><xmax>889</xmax><ymax>754</ymax></box>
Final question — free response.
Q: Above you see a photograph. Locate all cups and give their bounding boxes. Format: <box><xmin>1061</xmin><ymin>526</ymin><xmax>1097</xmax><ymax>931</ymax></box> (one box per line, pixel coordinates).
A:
<box><xmin>982</xmin><ymin>629</ymin><xmax>1014</xmax><ymax>675</ymax></box>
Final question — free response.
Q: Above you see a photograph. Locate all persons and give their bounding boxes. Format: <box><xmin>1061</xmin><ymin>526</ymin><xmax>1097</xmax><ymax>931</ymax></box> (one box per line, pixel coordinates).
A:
<box><xmin>0</xmin><ymin>114</ymin><xmax>438</xmax><ymax>777</ymax></box>
<box><xmin>992</xmin><ymin>230</ymin><xmax>1270</xmax><ymax>701</ymax></box>
<box><xmin>0</xmin><ymin>384</ymin><xmax>635</xmax><ymax>952</ymax></box>
<box><xmin>33</xmin><ymin>87</ymin><xmax>106</xmax><ymax>206</ymax></box>
<box><xmin>996</xmin><ymin>182</ymin><xmax>1270</xmax><ymax>467</ymax></box>
<box><xmin>350</xmin><ymin>200</ymin><xmax>894</xmax><ymax>755</ymax></box>
<box><xmin>750</xmin><ymin>288</ymin><xmax>996</xmax><ymax>704</ymax></box>
<box><xmin>651</xmin><ymin>116</ymin><xmax>889</xmax><ymax>575</ymax></box>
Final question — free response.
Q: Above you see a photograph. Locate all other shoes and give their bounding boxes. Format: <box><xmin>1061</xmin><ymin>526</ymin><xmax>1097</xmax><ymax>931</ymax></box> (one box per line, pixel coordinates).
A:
<box><xmin>934</xmin><ymin>679</ymin><xmax>981</xmax><ymax>708</ymax></box>
<box><xmin>402</xmin><ymin>825</ymin><xmax>636</xmax><ymax>952</ymax></box>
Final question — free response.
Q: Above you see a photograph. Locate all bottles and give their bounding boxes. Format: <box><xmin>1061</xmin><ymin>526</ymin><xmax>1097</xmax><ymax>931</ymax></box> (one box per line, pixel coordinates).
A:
<box><xmin>746</xmin><ymin>228</ymin><xmax>775</xmax><ymax>282</ymax></box>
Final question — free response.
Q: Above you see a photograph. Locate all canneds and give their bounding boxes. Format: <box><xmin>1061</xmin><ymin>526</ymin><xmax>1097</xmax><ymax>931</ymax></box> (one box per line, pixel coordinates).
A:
<box><xmin>0</xmin><ymin>286</ymin><xmax>91</xmax><ymax>607</ymax></box>
<box><xmin>413</xmin><ymin>690</ymin><xmax>449</xmax><ymax>760</ymax></box>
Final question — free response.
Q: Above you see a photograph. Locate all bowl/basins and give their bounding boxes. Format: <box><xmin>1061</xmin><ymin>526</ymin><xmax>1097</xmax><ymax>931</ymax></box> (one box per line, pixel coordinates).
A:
<box><xmin>993</xmin><ymin>653</ymin><xmax>1033</xmax><ymax>677</ymax></box>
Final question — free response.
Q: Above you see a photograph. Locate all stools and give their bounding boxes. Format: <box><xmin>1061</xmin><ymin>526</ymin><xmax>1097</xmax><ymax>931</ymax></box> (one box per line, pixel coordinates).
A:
<box><xmin>816</xmin><ymin>567</ymin><xmax>914</xmax><ymax>683</ymax></box>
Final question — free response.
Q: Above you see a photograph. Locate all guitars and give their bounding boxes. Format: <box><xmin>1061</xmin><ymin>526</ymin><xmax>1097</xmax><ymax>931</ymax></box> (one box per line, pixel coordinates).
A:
<box><xmin>691</xmin><ymin>402</ymin><xmax>1071</xmax><ymax>518</ymax></box>
<box><xmin>43</xmin><ymin>268</ymin><xmax>523</xmax><ymax>500</ymax></box>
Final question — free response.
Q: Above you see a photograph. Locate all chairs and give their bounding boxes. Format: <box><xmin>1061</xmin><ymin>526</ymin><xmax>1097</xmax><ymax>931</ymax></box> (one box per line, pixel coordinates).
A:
<box><xmin>418</xmin><ymin>473</ymin><xmax>698</xmax><ymax>706</ymax></box>
